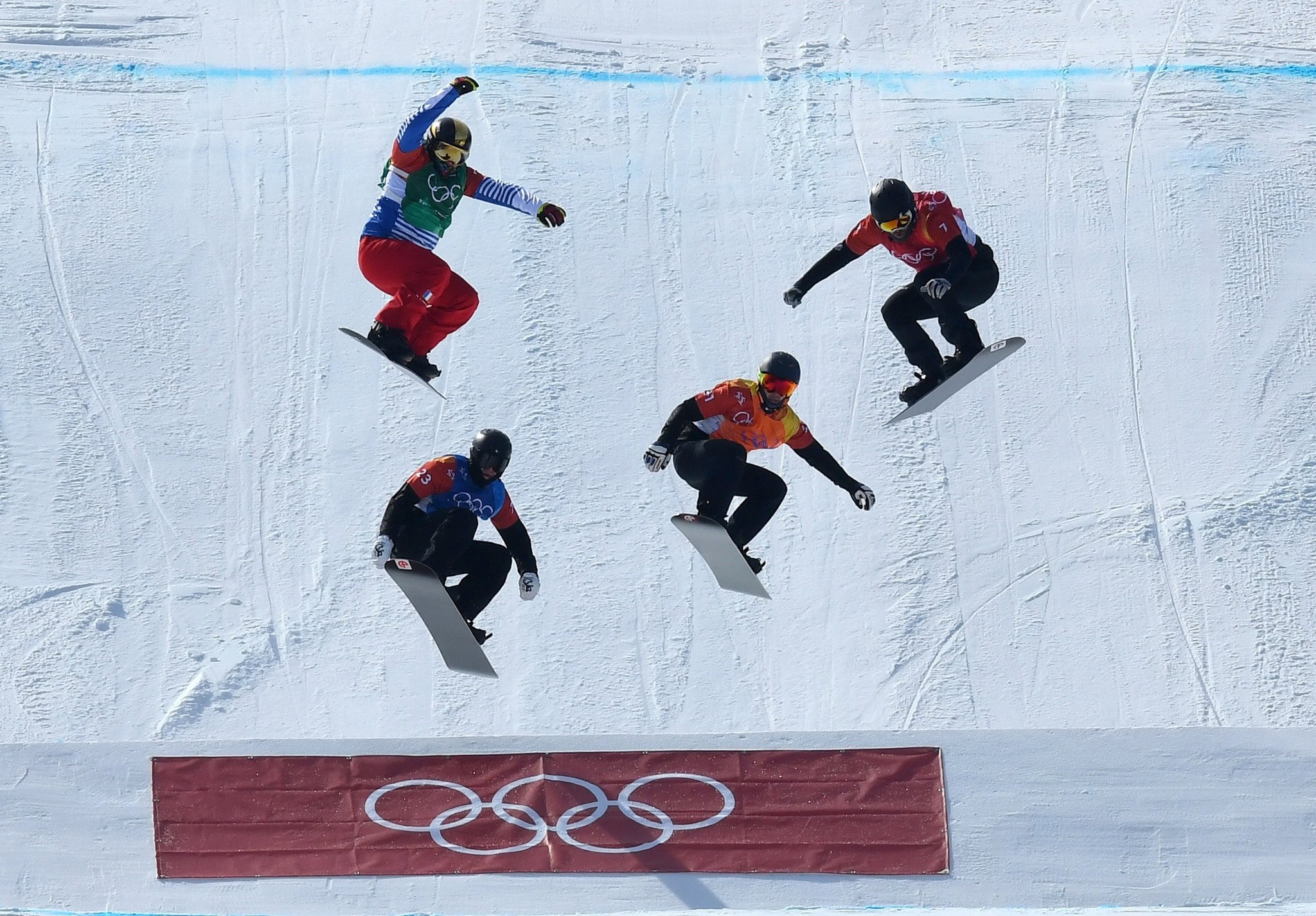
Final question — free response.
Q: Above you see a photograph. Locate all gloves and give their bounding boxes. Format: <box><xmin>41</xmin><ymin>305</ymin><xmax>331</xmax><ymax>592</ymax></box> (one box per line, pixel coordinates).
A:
<box><xmin>783</xmin><ymin>287</ymin><xmax>803</xmax><ymax>308</ymax></box>
<box><xmin>450</xmin><ymin>76</ymin><xmax>479</xmax><ymax>95</ymax></box>
<box><xmin>371</xmin><ymin>536</ymin><xmax>393</xmax><ymax>569</ymax></box>
<box><xmin>518</xmin><ymin>572</ymin><xmax>540</xmax><ymax>599</ymax></box>
<box><xmin>643</xmin><ymin>440</ymin><xmax>672</xmax><ymax>472</ymax></box>
<box><xmin>849</xmin><ymin>483</ymin><xmax>875</xmax><ymax>510</ymax></box>
<box><xmin>537</xmin><ymin>202</ymin><xmax>566</xmax><ymax>227</ymax></box>
<box><xmin>920</xmin><ymin>278</ymin><xmax>951</xmax><ymax>299</ymax></box>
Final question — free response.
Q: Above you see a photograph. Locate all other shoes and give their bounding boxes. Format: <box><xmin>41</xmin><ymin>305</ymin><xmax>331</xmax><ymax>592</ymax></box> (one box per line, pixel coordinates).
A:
<box><xmin>368</xmin><ymin>322</ymin><xmax>413</xmax><ymax>362</ymax></box>
<box><xmin>408</xmin><ymin>355</ymin><xmax>439</xmax><ymax>379</ymax></box>
<box><xmin>936</xmin><ymin>343</ymin><xmax>982</xmax><ymax>378</ymax></box>
<box><xmin>898</xmin><ymin>366</ymin><xmax>947</xmax><ymax>404</ymax></box>
<box><xmin>742</xmin><ymin>552</ymin><xmax>763</xmax><ymax>577</ymax></box>
<box><xmin>466</xmin><ymin>621</ymin><xmax>485</xmax><ymax>644</ymax></box>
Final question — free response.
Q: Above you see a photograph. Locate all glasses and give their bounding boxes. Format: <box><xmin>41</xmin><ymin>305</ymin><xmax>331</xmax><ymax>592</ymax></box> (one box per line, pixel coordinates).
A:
<box><xmin>434</xmin><ymin>142</ymin><xmax>469</xmax><ymax>166</ymax></box>
<box><xmin>476</xmin><ymin>451</ymin><xmax>508</xmax><ymax>473</ymax></box>
<box><xmin>761</xmin><ymin>374</ymin><xmax>794</xmax><ymax>397</ymax></box>
<box><xmin>879</xmin><ymin>213</ymin><xmax>912</xmax><ymax>233</ymax></box>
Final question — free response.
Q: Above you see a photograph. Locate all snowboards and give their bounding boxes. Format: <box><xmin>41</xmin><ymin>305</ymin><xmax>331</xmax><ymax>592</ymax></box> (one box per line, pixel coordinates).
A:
<box><xmin>386</xmin><ymin>560</ymin><xmax>498</xmax><ymax>679</ymax></box>
<box><xmin>338</xmin><ymin>326</ymin><xmax>451</xmax><ymax>399</ymax></box>
<box><xmin>670</xmin><ymin>515</ymin><xmax>775</xmax><ymax>601</ymax></box>
<box><xmin>883</xmin><ymin>337</ymin><xmax>1030</xmax><ymax>428</ymax></box>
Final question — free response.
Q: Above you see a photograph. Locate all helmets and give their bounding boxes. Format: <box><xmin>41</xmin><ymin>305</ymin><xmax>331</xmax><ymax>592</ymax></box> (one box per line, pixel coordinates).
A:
<box><xmin>869</xmin><ymin>178</ymin><xmax>914</xmax><ymax>222</ymax></box>
<box><xmin>471</xmin><ymin>428</ymin><xmax>512</xmax><ymax>486</ymax></box>
<box><xmin>428</xmin><ymin>117</ymin><xmax>471</xmax><ymax>175</ymax></box>
<box><xmin>760</xmin><ymin>351</ymin><xmax>800</xmax><ymax>384</ymax></box>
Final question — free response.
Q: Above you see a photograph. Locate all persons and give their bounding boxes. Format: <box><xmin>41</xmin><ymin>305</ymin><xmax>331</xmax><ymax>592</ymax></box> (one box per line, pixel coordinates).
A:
<box><xmin>372</xmin><ymin>429</ymin><xmax>540</xmax><ymax>645</ymax></box>
<box><xmin>357</xmin><ymin>76</ymin><xmax>567</xmax><ymax>385</ymax></box>
<box><xmin>641</xmin><ymin>353</ymin><xmax>876</xmax><ymax>577</ymax></box>
<box><xmin>782</xmin><ymin>179</ymin><xmax>1000</xmax><ymax>403</ymax></box>
